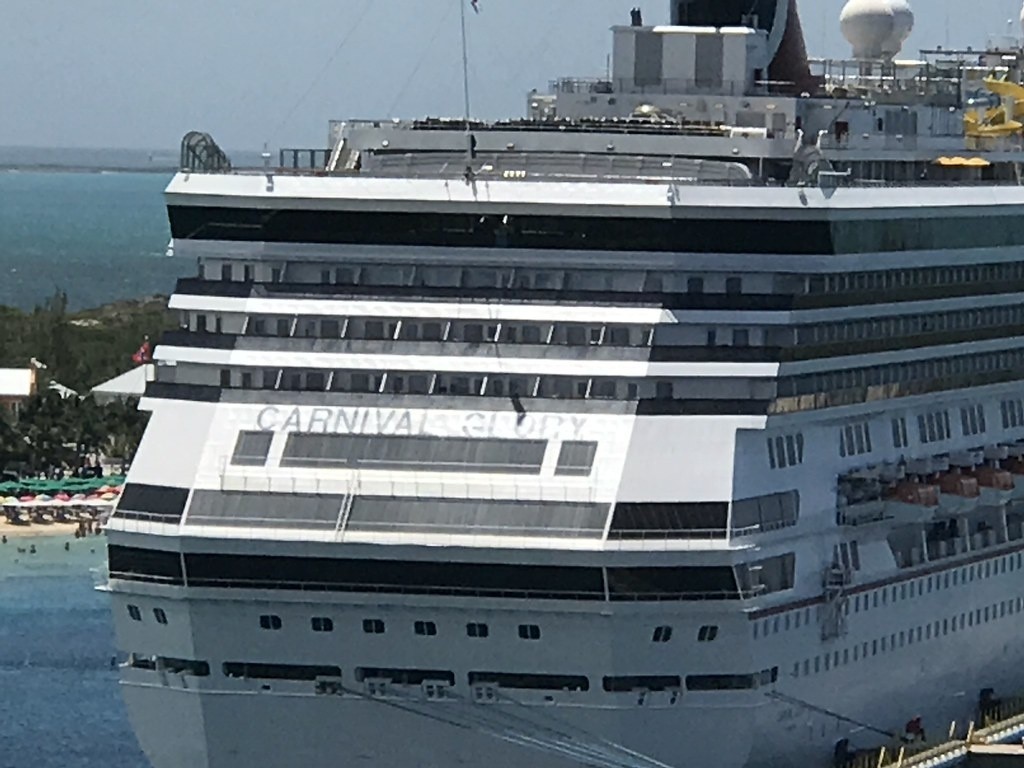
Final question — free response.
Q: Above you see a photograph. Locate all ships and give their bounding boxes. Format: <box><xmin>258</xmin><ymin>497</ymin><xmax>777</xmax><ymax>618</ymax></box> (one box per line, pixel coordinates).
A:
<box><xmin>88</xmin><ymin>1</ymin><xmax>1024</xmax><ymax>766</ymax></box>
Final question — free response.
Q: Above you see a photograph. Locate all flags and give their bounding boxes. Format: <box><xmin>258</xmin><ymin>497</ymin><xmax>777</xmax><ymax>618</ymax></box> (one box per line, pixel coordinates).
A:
<box><xmin>132</xmin><ymin>344</ymin><xmax>151</xmax><ymax>364</ymax></box>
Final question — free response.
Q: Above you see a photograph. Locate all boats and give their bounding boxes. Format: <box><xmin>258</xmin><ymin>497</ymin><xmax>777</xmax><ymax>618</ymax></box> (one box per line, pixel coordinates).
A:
<box><xmin>966</xmin><ymin>114</ymin><xmax>1021</xmax><ymax>151</ymax></box>
<box><xmin>1000</xmin><ymin>458</ymin><xmax>1024</xmax><ymax>502</ymax></box>
<box><xmin>890</xmin><ymin>483</ymin><xmax>939</xmax><ymax>524</ymax></box>
<box><xmin>975</xmin><ymin>469</ymin><xmax>1014</xmax><ymax>510</ymax></box>
<box><xmin>936</xmin><ymin>471</ymin><xmax>981</xmax><ymax>517</ymax></box>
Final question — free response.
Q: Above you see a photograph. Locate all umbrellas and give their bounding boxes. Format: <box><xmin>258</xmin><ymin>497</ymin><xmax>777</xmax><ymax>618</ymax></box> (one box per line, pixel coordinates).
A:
<box><xmin>0</xmin><ymin>473</ymin><xmax>125</xmax><ymax>510</ymax></box>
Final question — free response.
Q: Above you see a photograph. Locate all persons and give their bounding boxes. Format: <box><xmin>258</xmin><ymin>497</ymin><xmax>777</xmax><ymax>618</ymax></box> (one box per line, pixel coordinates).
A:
<box><xmin>5</xmin><ymin>508</ymin><xmax>68</xmax><ymax>525</ymax></box>
<box><xmin>2</xmin><ymin>536</ymin><xmax>7</xmax><ymax>543</ymax></box>
<box><xmin>65</xmin><ymin>542</ymin><xmax>69</xmax><ymax>551</ymax></box>
<box><xmin>75</xmin><ymin>515</ymin><xmax>108</xmax><ymax>538</ymax></box>
<box><xmin>31</xmin><ymin>544</ymin><xmax>36</xmax><ymax>553</ymax></box>
